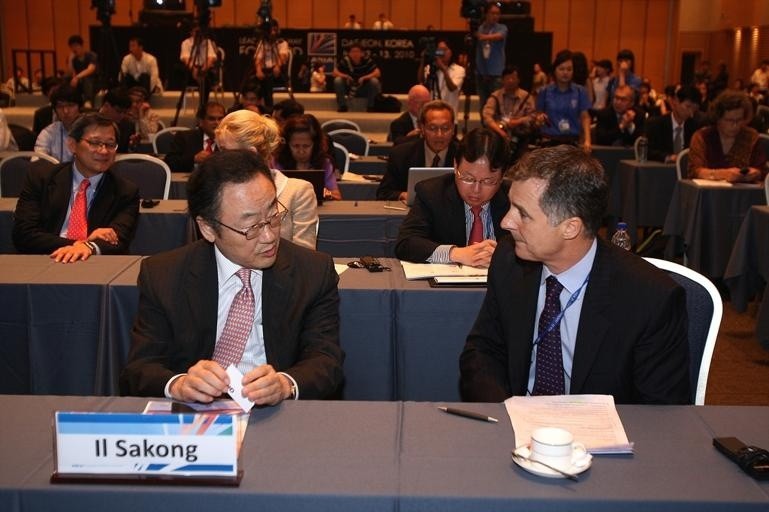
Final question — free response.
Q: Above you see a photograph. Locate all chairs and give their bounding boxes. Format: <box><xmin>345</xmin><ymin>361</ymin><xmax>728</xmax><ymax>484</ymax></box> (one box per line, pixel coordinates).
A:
<box><xmin>1</xmin><ymin>118</ymin><xmax>724</xmax><ymax>405</ymax></box>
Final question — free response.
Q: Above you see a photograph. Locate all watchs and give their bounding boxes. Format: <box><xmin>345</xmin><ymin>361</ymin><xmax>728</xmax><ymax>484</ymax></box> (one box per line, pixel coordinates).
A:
<box><xmin>283</xmin><ymin>375</ymin><xmax>297</xmax><ymax>399</ymax></box>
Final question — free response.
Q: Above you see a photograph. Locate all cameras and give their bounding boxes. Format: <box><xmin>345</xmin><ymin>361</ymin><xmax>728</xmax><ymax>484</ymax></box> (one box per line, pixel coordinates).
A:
<box><xmin>498</xmin><ymin>116</ymin><xmax>510</xmax><ymax>129</ymax></box>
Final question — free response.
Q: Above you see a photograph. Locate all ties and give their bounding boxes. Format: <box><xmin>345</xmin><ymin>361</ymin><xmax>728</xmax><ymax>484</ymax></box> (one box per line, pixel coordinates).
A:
<box><xmin>468</xmin><ymin>206</ymin><xmax>483</xmax><ymax>245</ymax></box>
<box><xmin>673</xmin><ymin>126</ymin><xmax>681</xmax><ymax>155</ymax></box>
<box><xmin>68</xmin><ymin>179</ymin><xmax>89</xmax><ymax>241</ymax></box>
<box><xmin>432</xmin><ymin>154</ymin><xmax>439</xmax><ymax>167</ymax></box>
<box><xmin>532</xmin><ymin>276</ymin><xmax>565</xmax><ymax>396</ymax></box>
<box><xmin>211</xmin><ymin>269</ymin><xmax>255</xmax><ymax>371</ymax></box>
<box><xmin>204</xmin><ymin>137</ymin><xmax>213</xmax><ymax>153</ymax></box>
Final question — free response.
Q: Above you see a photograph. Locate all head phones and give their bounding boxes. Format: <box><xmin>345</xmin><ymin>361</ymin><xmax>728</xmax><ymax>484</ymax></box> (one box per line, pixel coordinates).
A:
<box><xmin>272</xmin><ymin>19</ymin><xmax>281</xmax><ymax>36</ymax></box>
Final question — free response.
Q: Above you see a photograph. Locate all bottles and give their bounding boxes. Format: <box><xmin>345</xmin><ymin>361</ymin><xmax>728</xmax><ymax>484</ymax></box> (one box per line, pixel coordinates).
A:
<box><xmin>610</xmin><ymin>222</ymin><xmax>633</xmax><ymax>253</ymax></box>
<box><xmin>637</xmin><ymin>137</ymin><xmax>649</xmax><ymax>164</ymax></box>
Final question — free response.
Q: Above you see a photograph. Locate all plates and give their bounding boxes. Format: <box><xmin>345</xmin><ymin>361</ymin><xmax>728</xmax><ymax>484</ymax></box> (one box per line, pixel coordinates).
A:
<box><xmin>512</xmin><ymin>448</ymin><xmax>594</xmax><ymax>479</ymax></box>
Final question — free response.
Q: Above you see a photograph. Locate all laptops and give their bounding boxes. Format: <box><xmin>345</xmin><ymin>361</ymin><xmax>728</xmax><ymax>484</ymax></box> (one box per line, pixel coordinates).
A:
<box><xmin>401</xmin><ymin>167</ymin><xmax>455</xmax><ymax>206</ymax></box>
<box><xmin>274</xmin><ymin>169</ymin><xmax>326</xmax><ymax>207</ymax></box>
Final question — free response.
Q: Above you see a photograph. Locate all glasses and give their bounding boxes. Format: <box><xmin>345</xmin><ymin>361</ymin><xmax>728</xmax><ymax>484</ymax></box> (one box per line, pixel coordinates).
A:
<box><xmin>456</xmin><ymin>164</ymin><xmax>502</xmax><ymax>186</ymax></box>
<box><xmin>72</xmin><ymin>133</ymin><xmax>119</xmax><ymax>154</ymax></box>
<box><xmin>200</xmin><ymin>200</ymin><xmax>289</xmax><ymax>242</ymax></box>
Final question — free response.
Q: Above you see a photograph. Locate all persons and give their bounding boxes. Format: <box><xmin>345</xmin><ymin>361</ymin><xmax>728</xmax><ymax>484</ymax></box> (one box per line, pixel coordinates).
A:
<box><xmin>120</xmin><ymin>148</ymin><xmax>348</xmax><ymax>406</ymax></box>
<box><xmin>584</xmin><ymin>46</ymin><xmax>769</xmax><ymax>183</ymax></box>
<box><xmin>460</xmin><ymin>143</ymin><xmax>691</xmax><ymax>402</ymax></box>
<box><xmin>1</xmin><ymin>6</ymin><xmax>593</xmax><ymax>270</ymax></box>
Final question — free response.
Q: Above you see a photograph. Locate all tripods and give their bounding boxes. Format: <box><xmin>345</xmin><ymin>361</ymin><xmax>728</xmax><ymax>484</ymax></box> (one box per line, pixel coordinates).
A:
<box><xmin>423</xmin><ymin>72</ymin><xmax>442</xmax><ymax>100</ymax></box>
<box><xmin>171</xmin><ymin>27</ymin><xmax>239</xmax><ymax>127</ymax></box>
<box><xmin>237</xmin><ymin>22</ymin><xmax>295</xmax><ymax>101</ymax></box>
<box><xmin>454</xmin><ymin>39</ymin><xmax>487</xmax><ymax>134</ymax></box>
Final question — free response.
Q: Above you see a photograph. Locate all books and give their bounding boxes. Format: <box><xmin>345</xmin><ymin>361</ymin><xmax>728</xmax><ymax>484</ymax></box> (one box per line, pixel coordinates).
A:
<box><xmin>504</xmin><ymin>394</ymin><xmax>634</xmax><ymax>458</ymax></box>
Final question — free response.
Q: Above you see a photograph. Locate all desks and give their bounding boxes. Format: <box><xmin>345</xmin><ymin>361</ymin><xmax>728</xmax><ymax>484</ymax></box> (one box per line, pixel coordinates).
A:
<box><xmin>724</xmin><ymin>204</ymin><xmax>769</xmax><ymax>355</ymax></box>
<box><xmin>1</xmin><ymin>391</ymin><xmax>768</xmax><ymax>512</ymax></box>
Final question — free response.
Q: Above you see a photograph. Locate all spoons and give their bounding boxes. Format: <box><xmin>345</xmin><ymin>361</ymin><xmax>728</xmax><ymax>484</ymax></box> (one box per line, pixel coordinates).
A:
<box><xmin>511</xmin><ymin>450</ymin><xmax>579</xmax><ymax>482</ymax></box>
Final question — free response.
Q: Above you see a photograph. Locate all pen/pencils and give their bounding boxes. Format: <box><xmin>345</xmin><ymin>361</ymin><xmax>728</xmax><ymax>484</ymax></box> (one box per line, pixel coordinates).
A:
<box><xmin>438</xmin><ymin>407</ymin><xmax>498</xmax><ymax>422</ymax></box>
<box><xmin>384</xmin><ymin>206</ymin><xmax>406</xmax><ymax>211</ymax></box>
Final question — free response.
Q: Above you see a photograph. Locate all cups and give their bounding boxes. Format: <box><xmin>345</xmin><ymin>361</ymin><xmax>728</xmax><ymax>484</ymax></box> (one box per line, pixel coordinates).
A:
<box><xmin>529</xmin><ymin>427</ymin><xmax>585</xmax><ymax>475</ymax></box>
